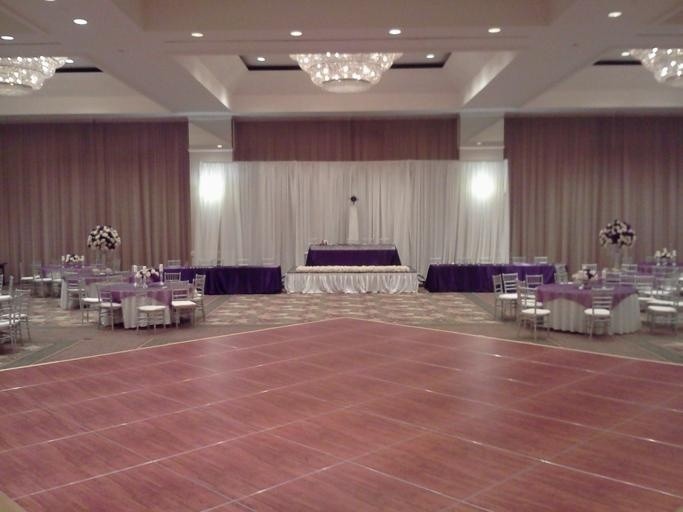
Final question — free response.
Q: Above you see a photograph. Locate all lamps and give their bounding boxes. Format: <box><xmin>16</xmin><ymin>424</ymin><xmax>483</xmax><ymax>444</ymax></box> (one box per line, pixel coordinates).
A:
<box><xmin>627</xmin><ymin>47</ymin><xmax>682</xmax><ymax>89</ymax></box>
<box><xmin>288</xmin><ymin>52</ymin><xmax>408</xmax><ymax>93</ymax></box>
<box><xmin>0</xmin><ymin>56</ymin><xmax>69</xmax><ymax>97</ymax></box>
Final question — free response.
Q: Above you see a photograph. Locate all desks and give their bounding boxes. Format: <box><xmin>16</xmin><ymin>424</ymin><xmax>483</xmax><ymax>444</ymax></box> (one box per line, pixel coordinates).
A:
<box><xmin>422</xmin><ymin>262</ymin><xmax>567</xmax><ymax>293</ymax></box>
<box><xmin>155</xmin><ymin>265</ymin><xmax>283</xmax><ymax>294</ymax></box>
<box><xmin>304</xmin><ymin>244</ymin><xmax>401</xmax><ymax>265</ymax></box>
<box><xmin>283</xmin><ymin>265</ymin><xmax>418</xmax><ymax>294</ymax></box>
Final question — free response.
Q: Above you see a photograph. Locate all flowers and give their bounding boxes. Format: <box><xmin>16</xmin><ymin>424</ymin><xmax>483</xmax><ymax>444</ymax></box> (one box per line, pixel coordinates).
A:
<box><xmin>84</xmin><ymin>224</ymin><xmax>120</xmax><ymax>251</ymax></box>
<box><xmin>596</xmin><ymin>217</ymin><xmax>640</xmax><ymax>252</ymax></box>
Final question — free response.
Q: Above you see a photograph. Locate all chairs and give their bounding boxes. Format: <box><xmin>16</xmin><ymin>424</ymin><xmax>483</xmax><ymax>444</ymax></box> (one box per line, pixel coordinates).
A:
<box><xmin>492</xmin><ymin>253</ymin><xmax>682</xmax><ymax>339</ymax></box>
<box><xmin>0</xmin><ymin>252</ymin><xmax>208</xmax><ymax>354</ymax></box>
